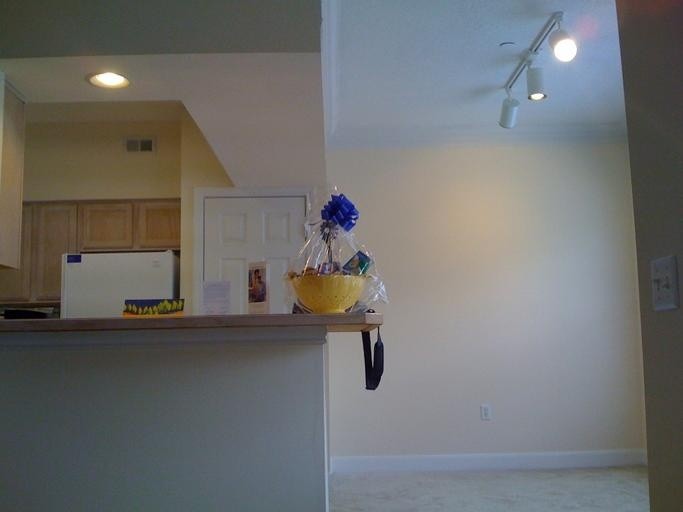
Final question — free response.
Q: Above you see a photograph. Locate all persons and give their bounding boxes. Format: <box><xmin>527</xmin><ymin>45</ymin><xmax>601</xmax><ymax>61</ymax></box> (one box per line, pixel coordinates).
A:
<box><xmin>250</xmin><ymin>269</ymin><xmax>265</xmax><ymax>301</ymax></box>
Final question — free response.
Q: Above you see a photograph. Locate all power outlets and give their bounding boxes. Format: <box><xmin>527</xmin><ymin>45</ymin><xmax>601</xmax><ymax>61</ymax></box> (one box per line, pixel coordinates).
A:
<box><xmin>650</xmin><ymin>254</ymin><xmax>679</xmax><ymax>312</ymax></box>
<box><xmin>481</xmin><ymin>405</ymin><xmax>491</xmax><ymax>419</ymax></box>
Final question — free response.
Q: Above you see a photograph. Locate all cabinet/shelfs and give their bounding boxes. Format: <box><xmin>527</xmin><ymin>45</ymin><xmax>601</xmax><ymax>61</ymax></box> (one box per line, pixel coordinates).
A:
<box><xmin>0</xmin><ymin>201</ymin><xmax>181</xmax><ymax>309</ymax></box>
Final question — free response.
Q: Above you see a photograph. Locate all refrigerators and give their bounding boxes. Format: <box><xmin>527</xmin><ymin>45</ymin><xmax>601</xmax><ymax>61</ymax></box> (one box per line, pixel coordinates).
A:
<box><xmin>59</xmin><ymin>248</ymin><xmax>179</xmax><ymax>322</ymax></box>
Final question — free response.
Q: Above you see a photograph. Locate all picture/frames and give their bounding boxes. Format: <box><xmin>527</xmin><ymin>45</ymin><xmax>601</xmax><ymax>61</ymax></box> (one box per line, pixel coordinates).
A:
<box><xmin>247</xmin><ymin>260</ymin><xmax>271</xmax><ymax>312</ymax></box>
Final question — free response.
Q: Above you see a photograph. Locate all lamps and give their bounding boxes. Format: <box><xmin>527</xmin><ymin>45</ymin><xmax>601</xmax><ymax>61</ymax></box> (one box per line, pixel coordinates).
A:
<box><xmin>498</xmin><ymin>12</ymin><xmax>578</xmax><ymax>129</ymax></box>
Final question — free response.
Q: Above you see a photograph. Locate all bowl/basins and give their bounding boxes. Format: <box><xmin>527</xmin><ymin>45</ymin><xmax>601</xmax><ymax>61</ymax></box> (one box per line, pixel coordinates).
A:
<box><xmin>289</xmin><ymin>273</ymin><xmax>368</xmax><ymax>313</ymax></box>
